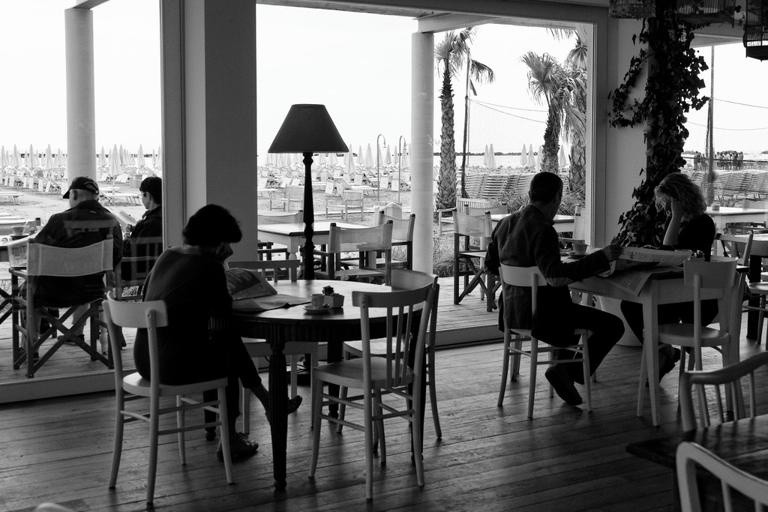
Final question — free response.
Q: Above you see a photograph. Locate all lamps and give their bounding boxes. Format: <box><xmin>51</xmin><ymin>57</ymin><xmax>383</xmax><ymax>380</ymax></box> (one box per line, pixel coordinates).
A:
<box><xmin>269</xmin><ymin>104</ymin><xmax>351</xmax><ymax>278</ymax></box>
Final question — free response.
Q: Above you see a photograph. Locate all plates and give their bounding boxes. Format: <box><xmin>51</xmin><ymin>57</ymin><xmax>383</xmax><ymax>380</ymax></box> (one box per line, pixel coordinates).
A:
<box><xmin>569</xmin><ymin>252</ymin><xmax>587</xmax><ymax>259</ymax></box>
<box><xmin>10</xmin><ymin>235</ymin><xmax>28</xmax><ymax>240</ymax></box>
<box><xmin>303</xmin><ymin>304</ymin><xmax>331</xmax><ymax>314</ymax></box>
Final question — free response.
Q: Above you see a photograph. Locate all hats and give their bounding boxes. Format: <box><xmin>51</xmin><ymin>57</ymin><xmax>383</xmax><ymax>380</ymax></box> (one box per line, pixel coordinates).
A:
<box><xmin>64</xmin><ymin>176</ymin><xmax>98</xmax><ymax>198</ymax></box>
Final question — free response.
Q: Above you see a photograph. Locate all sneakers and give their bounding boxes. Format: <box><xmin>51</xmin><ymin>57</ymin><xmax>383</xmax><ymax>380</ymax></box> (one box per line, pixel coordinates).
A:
<box><xmin>644</xmin><ymin>346</ymin><xmax>682</xmax><ymax>388</ymax></box>
<box><xmin>265</xmin><ymin>395</ymin><xmax>302</xmax><ymax>425</ymax></box>
<box><xmin>217</xmin><ymin>432</ymin><xmax>259</xmax><ymax>463</ymax></box>
<box><xmin>545</xmin><ymin>368</ymin><xmax>582</xmax><ymax>405</ymax></box>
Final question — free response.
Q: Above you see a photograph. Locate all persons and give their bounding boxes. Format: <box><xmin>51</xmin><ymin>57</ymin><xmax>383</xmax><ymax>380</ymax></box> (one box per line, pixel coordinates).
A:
<box><xmin>134</xmin><ymin>204</ymin><xmax>302</xmax><ymax>462</ymax></box>
<box><xmin>14</xmin><ymin>176</ymin><xmax>122</xmax><ymax>364</ymax></box>
<box><xmin>484</xmin><ymin>171</ymin><xmax>622</xmax><ymax>406</ymax></box>
<box><xmin>121</xmin><ymin>177</ymin><xmax>162</xmax><ymax>281</ymax></box>
<box><xmin>621</xmin><ymin>172</ymin><xmax>719</xmax><ymax>388</ymax></box>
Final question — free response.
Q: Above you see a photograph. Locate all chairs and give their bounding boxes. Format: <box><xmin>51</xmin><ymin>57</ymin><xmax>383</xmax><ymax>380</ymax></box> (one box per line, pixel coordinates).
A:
<box><xmin>496</xmin><ymin>263</ymin><xmax>595</xmax><ymax>418</ymax></box>
<box><xmin>679</xmin><ymin>350</ymin><xmax>767</xmax><ymax>431</ymax></box>
<box><xmin>337</xmin><ymin>270</ymin><xmax>447</xmax><ymax>457</ymax></box>
<box><xmin>309</xmin><ymin>281</ymin><xmax>435</xmax><ymax>499</ymax></box>
<box><xmin>0</xmin><ymin>196</ymin><xmax>766</xmax><ymax>376</ymax></box>
<box><xmin>676</xmin><ymin>443</ymin><xmax>766</xmax><ymax>511</ymax></box>
<box><xmin>104</xmin><ymin>291</ymin><xmax>240</xmax><ymax>510</ymax></box>
<box><xmin>633</xmin><ymin>262</ymin><xmax>734</xmax><ymax>425</ymax></box>
<box><xmin>657</xmin><ymin>232</ymin><xmax>753</xmax><ymax>417</ymax></box>
<box><xmin>233</xmin><ymin>258</ymin><xmax>318</xmax><ymax>429</ymax></box>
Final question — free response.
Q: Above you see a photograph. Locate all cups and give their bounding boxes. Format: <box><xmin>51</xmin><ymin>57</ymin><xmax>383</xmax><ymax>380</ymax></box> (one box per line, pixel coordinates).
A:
<box><xmin>311</xmin><ymin>293</ymin><xmax>325</xmax><ymax>309</ymax></box>
<box><xmin>11</xmin><ymin>226</ymin><xmax>25</xmax><ymax>236</ymax></box>
<box><xmin>571</xmin><ymin>242</ymin><xmax>589</xmax><ymax>254</ymax></box>
